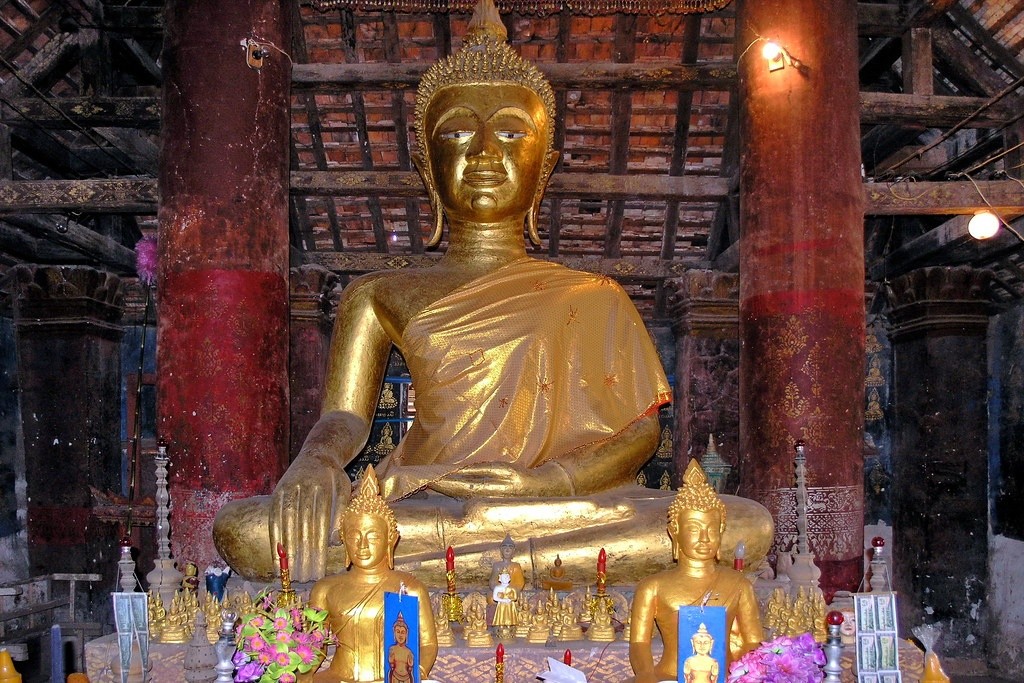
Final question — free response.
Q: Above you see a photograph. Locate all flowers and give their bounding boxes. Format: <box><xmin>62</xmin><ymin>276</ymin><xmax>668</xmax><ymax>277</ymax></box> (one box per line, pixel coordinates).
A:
<box><xmin>726</xmin><ymin>632</ymin><xmax>827</xmax><ymax>683</ymax></box>
<box><xmin>234</xmin><ymin>582</ymin><xmax>355</xmax><ymax>683</ymax></box>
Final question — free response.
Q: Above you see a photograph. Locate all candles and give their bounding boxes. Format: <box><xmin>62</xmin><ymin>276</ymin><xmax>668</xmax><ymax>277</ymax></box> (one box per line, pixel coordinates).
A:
<box><xmin>597</xmin><ymin>547</ymin><xmax>607</xmax><ymax>588</ymax></box>
<box><xmin>495</xmin><ymin>643</ymin><xmax>504</xmax><ymax>683</ymax></box>
<box><xmin>563</xmin><ymin>649</ymin><xmax>571</xmax><ymax>667</ymax></box>
<box><xmin>446</xmin><ymin>546</ymin><xmax>456</xmax><ymax>592</ymax></box>
<box><xmin>277</xmin><ymin>542</ymin><xmax>291</xmax><ymax>589</ymax></box>
<box><xmin>734</xmin><ymin>539</ymin><xmax>745</xmax><ymax>571</ymax></box>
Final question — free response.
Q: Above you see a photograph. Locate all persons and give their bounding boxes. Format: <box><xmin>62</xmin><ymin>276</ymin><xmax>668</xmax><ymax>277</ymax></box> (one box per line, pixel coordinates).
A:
<box><xmin>377</xmin><ymin>375</ymin><xmax>397</xmax><ymax>409</ymax></box>
<box><xmin>387</xmin><ymin>611</ymin><xmax>415</xmax><ymax>683</ymax></box>
<box><xmin>764</xmin><ymin>583</ymin><xmax>855</xmax><ymax>666</ymax></box>
<box><xmin>147</xmin><ymin>561</ymin><xmax>309</xmax><ymax>645</ymax></box>
<box><xmin>292</xmin><ymin>463</ymin><xmax>440</xmax><ymax>683</ymax></box>
<box><xmin>374</xmin><ymin>421</ymin><xmax>396</xmax><ymax>455</ymax></box>
<box><xmin>429</xmin><ymin>530</ymin><xmax>635</xmax><ymax>648</ymax></box>
<box><xmin>617</xmin><ymin>458</ymin><xmax>764</xmax><ymax>683</ymax></box>
<box><xmin>656</xmin><ymin>422</ymin><xmax>673</xmax><ymax>458</ymax></box>
<box><xmin>659</xmin><ymin>469</ymin><xmax>671</xmax><ymax>491</ymax></box>
<box><xmin>206</xmin><ymin>0</ymin><xmax>776</xmax><ymax>584</ymax></box>
<box><xmin>681</xmin><ymin>622</ymin><xmax>720</xmax><ymax>683</ymax></box>
<box><xmin>863</xmin><ymin>323</ymin><xmax>886</xmax><ymax>420</ymax></box>
<box><xmin>864</xmin><ymin>431</ymin><xmax>876</xmax><ymax>450</ymax></box>
<box><xmin>636</xmin><ymin>470</ymin><xmax>647</xmax><ymax>488</ymax></box>
